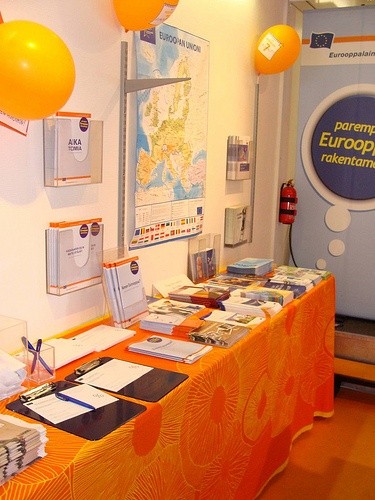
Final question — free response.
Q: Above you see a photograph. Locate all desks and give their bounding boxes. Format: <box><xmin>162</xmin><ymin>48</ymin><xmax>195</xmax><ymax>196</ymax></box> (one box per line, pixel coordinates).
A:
<box><xmin>0</xmin><ymin>255</ymin><xmax>339</xmax><ymax>500</ymax></box>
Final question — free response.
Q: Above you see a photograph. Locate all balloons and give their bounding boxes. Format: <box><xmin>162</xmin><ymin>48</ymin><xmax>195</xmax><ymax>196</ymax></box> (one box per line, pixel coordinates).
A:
<box><xmin>112</xmin><ymin>0</ymin><xmax>178</xmax><ymax>33</ymax></box>
<box><xmin>0</xmin><ymin>20</ymin><xmax>76</xmax><ymax>122</ymax></box>
<box><xmin>254</xmin><ymin>24</ymin><xmax>301</xmax><ymax>75</ymax></box>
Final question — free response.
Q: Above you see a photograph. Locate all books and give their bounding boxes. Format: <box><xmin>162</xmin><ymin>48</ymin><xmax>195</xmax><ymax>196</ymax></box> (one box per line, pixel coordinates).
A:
<box><xmin>0</xmin><ymin>413</ymin><xmax>48</xmax><ymax>487</ymax></box>
<box><xmin>25</xmin><ymin>112</ymin><xmax>332</xmax><ymax>372</ymax></box>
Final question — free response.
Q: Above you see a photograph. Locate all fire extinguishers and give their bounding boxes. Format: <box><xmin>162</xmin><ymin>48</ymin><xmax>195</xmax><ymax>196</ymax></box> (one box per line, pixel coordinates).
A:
<box><xmin>278</xmin><ymin>179</ymin><xmax>298</xmax><ymax>225</ymax></box>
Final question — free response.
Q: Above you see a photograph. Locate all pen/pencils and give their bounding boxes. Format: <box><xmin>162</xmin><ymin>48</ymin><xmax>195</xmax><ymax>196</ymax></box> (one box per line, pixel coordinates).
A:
<box><xmin>56</xmin><ymin>391</ymin><xmax>97</xmax><ymax>411</ymax></box>
<box><xmin>31</xmin><ymin>339</ymin><xmax>42</xmax><ymax>375</ymax></box>
<box><xmin>20</xmin><ymin>336</ymin><xmax>54</xmax><ymax>376</ymax></box>
